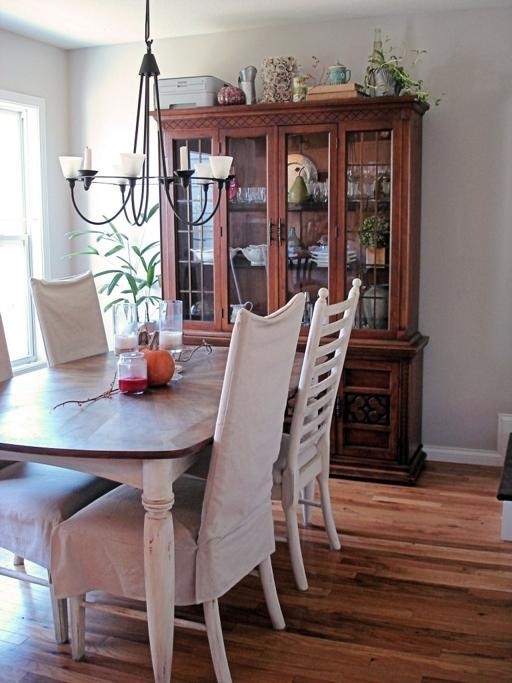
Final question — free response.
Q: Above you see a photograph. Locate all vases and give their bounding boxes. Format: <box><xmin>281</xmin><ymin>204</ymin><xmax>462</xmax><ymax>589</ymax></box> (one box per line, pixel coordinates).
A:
<box><xmin>362</xmin><ymin>283</ymin><xmax>389</xmax><ymax>329</ymax></box>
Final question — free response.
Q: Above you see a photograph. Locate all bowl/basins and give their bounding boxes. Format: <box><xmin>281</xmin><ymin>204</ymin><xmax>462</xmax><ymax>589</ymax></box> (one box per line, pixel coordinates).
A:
<box><xmin>190</xmin><ymin>244</ymin><xmax>267</xmax><ymax>266</ymax></box>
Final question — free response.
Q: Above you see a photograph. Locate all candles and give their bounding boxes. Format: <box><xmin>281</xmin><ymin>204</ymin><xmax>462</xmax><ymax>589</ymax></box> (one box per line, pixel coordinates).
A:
<box><xmin>159</xmin><ymin>330</ymin><xmax>182</xmax><ymax>350</ymax></box>
<box><xmin>115</xmin><ymin>332</ymin><xmax>139</xmax><ymax>354</ymax></box>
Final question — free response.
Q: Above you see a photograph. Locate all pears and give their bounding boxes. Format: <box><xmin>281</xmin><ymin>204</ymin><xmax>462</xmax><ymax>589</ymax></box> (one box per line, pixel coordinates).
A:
<box><xmin>288</xmin><ymin>165</ymin><xmax>309</xmax><ymax>204</ymax></box>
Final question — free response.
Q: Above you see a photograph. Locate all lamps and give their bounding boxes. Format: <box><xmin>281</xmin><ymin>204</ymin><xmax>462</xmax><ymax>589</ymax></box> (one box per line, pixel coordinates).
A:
<box><xmin>59</xmin><ymin>1</ymin><xmax>237</xmax><ymax>227</ymax></box>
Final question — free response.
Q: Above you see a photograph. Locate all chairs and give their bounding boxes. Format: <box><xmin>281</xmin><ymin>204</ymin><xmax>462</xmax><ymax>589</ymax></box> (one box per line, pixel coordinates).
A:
<box><xmin>30</xmin><ymin>270</ymin><xmax>109</xmax><ymax>368</ymax></box>
<box><xmin>48</xmin><ymin>292</ymin><xmax>307</xmax><ymax>683</ymax></box>
<box><xmin>0</xmin><ymin>312</ymin><xmax>117</xmax><ymax>644</ymax></box>
<box><xmin>183</xmin><ymin>278</ymin><xmax>362</xmax><ymax>591</ymax></box>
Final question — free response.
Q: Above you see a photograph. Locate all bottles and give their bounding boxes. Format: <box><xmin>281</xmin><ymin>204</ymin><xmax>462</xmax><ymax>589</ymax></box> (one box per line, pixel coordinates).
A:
<box><xmin>226</xmin><ymin>168</ymin><xmax>238</xmax><ymax>204</ymax></box>
<box><xmin>370</xmin><ymin>28</ymin><xmax>384</xmax><ymax>97</ymax></box>
<box><xmin>304</xmin><ymin>292</ymin><xmax>313</xmax><ymax>323</ymax></box>
<box><xmin>117</xmin><ymin>352</ymin><xmax>147</xmax><ymax>396</ymax></box>
<box><xmin>286</xmin><ymin>227</ymin><xmax>302</xmax><ymax>260</ymax></box>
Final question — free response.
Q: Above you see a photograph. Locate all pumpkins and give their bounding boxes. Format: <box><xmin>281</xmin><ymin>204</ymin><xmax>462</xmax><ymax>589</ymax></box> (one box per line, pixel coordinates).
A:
<box><xmin>138</xmin><ymin>330</ymin><xmax>175</xmax><ymax>388</ymax></box>
<box><xmin>216</xmin><ymin>83</ymin><xmax>246</xmax><ymax>105</ymax></box>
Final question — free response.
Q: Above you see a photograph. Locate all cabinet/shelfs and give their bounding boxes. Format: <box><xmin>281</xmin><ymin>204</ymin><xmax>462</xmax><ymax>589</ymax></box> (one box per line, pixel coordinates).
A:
<box><xmin>148</xmin><ymin>95</ymin><xmax>431</xmax><ymax>486</ymax></box>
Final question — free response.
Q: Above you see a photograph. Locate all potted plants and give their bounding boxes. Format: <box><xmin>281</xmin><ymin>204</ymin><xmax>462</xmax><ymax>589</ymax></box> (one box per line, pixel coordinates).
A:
<box><xmin>61</xmin><ymin>202</ymin><xmax>163</xmax><ymax>334</ymax></box>
<box><xmin>358</xmin><ymin>216</ymin><xmax>390</xmax><ymax>268</ymax></box>
<box><xmin>363</xmin><ymin>33</ymin><xmax>446</xmax><ymax>106</ymax></box>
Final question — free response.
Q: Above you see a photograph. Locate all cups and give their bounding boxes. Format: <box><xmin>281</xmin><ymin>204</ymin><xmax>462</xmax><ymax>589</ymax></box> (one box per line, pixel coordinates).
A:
<box><xmin>114</xmin><ymin>303</ymin><xmax>139</xmax><ymax>358</ymax></box>
<box><xmin>230</xmin><ymin>301</ymin><xmax>253</xmax><ymax>323</ymax></box>
<box><xmin>159</xmin><ymin>300</ymin><xmax>183</xmax><ymax>354</ymax></box>
<box><xmin>327</xmin><ymin>60</ymin><xmax>351</xmax><ymax>85</ymax></box>
<box><xmin>238</xmin><ymin>186</ymin><xmax>267</xmax><ymax>203</ymax></box>
<box><xmin>312</xmin><ymin>183</ymin><xmax>328</xmax><ymax>203</ymax></box>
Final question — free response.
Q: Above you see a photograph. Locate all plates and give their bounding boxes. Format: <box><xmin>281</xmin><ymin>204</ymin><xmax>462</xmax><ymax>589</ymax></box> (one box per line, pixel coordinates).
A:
<box><xmin>286</xmin><ymin>154</ymin><xmax>319</xmax><ymax>202</ymax></box>
<box><xmin>309</xmin><ymin>246</ymin><xmax>357</xmax><ymax>268</ymax></box>
